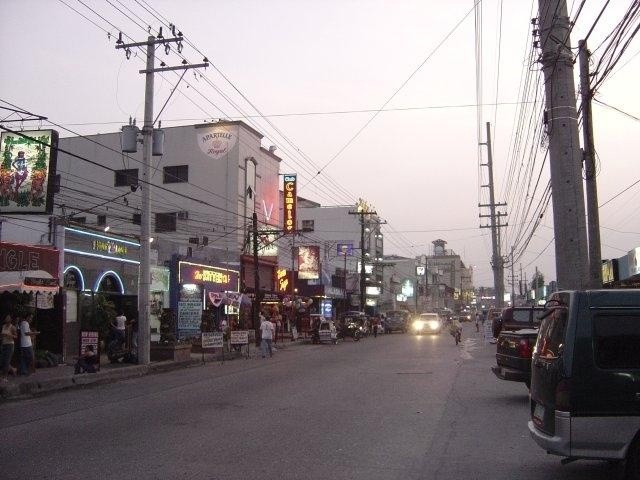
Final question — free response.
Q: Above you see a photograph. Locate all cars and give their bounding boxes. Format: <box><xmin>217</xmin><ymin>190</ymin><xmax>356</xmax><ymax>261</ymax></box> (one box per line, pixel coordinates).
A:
<box><xmin>417</xmin><ymin>313</ymin><xmax>444</xmax><ymax>335</ymax></box>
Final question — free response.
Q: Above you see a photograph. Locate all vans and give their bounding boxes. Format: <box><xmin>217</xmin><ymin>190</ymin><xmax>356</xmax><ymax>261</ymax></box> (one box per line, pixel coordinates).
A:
<box><xmin>524</xmin><ymin>287</ymin><xmax>640</xmax><ymax>478</ymax></box>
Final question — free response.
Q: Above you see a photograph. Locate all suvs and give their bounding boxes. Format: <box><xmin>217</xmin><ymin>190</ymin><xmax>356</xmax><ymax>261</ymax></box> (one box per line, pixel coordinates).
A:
<box><xmin>491</xmin><ymin>305</ymin><xmax>544</xmax><ymax>389</ymax></box>
<box><xmin>384</xmin><ymin>309</ymin><xmax>412</xmax><ymax>334</ymax></box>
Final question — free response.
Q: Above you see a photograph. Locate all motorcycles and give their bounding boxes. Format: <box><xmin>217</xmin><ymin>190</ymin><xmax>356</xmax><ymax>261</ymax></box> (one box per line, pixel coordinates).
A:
<box><xmin>452</xmin><ymin>327</ymin><xmax>462</xmax><ymax>345</ymax></box>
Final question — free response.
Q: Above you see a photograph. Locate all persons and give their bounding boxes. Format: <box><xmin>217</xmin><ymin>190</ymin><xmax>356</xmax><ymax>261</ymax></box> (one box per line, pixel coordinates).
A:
<box><xmin>277</xmin><ymin>307</ymin><xmax>482</xmax><ymax>346</ymax></box>
<box><xmin>1</xmin><ymin>304</ymin><xmax>277</xmax><ymax>383</ymax></box>
<box><xmin>12</xmin><ymin>149</ymin><xmax>33</xmax><ymax>191</ymax></box>
